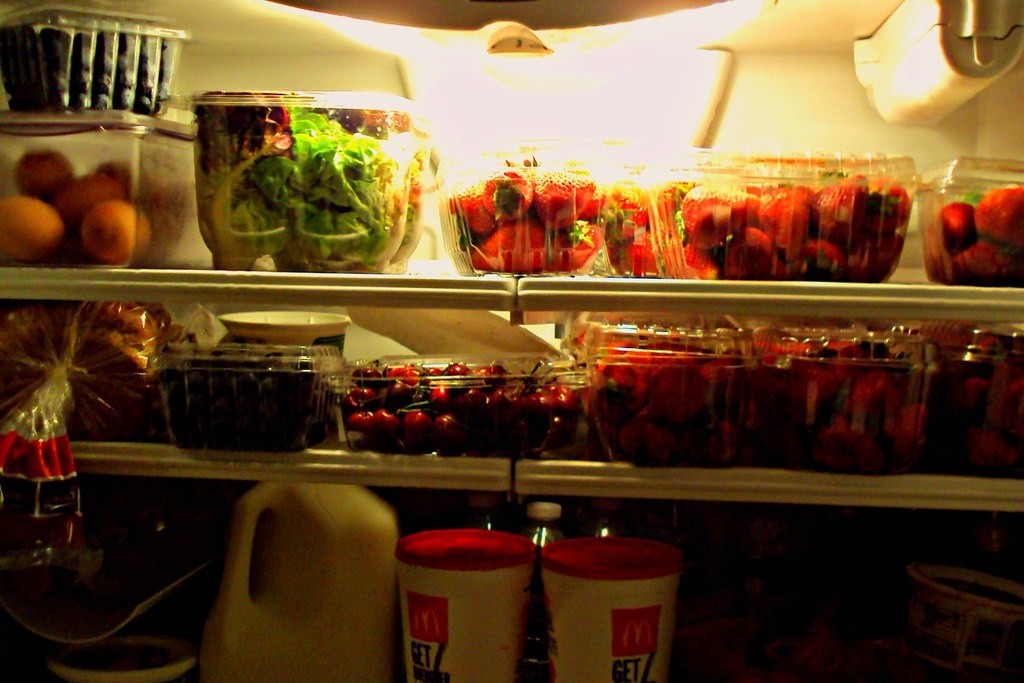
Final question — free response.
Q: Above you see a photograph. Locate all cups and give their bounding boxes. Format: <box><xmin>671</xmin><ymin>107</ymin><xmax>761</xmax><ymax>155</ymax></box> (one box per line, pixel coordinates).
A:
<box><xmin>396</xmin><ymin>529</ymin><xmax>536</xmax><ymax>683</ymax></box>
<box><xmin>542</xmin><ymin>538</ymin><xmax>678</xmax><ymax>683</ymax></box>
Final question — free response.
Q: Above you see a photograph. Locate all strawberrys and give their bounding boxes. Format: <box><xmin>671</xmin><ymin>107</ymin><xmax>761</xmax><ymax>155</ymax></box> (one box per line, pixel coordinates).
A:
<box><xmin>569</xmin><ymin>318</ymin><xmax>1024</xmax><ymax>480</ymax></box>
<box><xmin>445</xmin><ymin>158</ymin><xmax>1024</xmax><ymax>288</ymax></box>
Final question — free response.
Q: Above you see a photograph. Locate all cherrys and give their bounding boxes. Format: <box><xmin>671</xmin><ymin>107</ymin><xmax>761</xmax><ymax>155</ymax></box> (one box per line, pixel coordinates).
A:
<box><xmin>340</xmin><ymin>361</ymin><xmax>580</xmax><ymax>457</ymax></box>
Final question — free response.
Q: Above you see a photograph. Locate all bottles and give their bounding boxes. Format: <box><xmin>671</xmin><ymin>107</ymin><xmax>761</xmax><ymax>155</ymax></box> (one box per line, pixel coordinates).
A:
<box><xmin>472</xmin><ymin>496</ymin><xmax>633</xmax><ymax>682</ymax></box>
<box><xmin>204</xmin><ymin>478</ymin><xmax>398</xmax><ymax>683</ymax></box>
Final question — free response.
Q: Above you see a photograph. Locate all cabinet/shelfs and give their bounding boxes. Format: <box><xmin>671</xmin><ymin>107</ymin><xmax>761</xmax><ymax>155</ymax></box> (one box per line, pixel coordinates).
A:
<box><xmin>0</xmin><ymin>265</ymin><xmax>1023</xmax><ymax>529</ymax></box>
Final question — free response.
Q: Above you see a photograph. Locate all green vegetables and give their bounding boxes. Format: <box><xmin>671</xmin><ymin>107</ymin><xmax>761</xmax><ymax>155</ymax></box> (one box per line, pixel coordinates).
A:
<box><xmin>193</xmin><ymin>92</ymin><xmax>436</xmax><ymax>271</ymax></box>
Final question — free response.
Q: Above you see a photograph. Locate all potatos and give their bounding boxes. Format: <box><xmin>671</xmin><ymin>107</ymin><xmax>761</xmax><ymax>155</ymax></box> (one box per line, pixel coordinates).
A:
<box><xmin>0</xmin><ymin>149</ymin><xmax>182</xmax><ymax>265</ymax></box>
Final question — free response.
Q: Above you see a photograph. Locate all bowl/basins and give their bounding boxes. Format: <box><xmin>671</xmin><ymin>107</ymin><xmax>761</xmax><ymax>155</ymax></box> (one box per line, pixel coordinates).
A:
<box><xmin>905</xmin><ymin>561</ymin><xmax>1024</xmax><ymax>680</ymax></box>
<box><xmin>48</xmin><ymin>635</ymin><xmax>200</xmax><ymax>683</ymax></box>
<box><xmin>0</xmin><ymin>1</ymin><xmax>1023</xmax><ymax>477</ymax></box>
